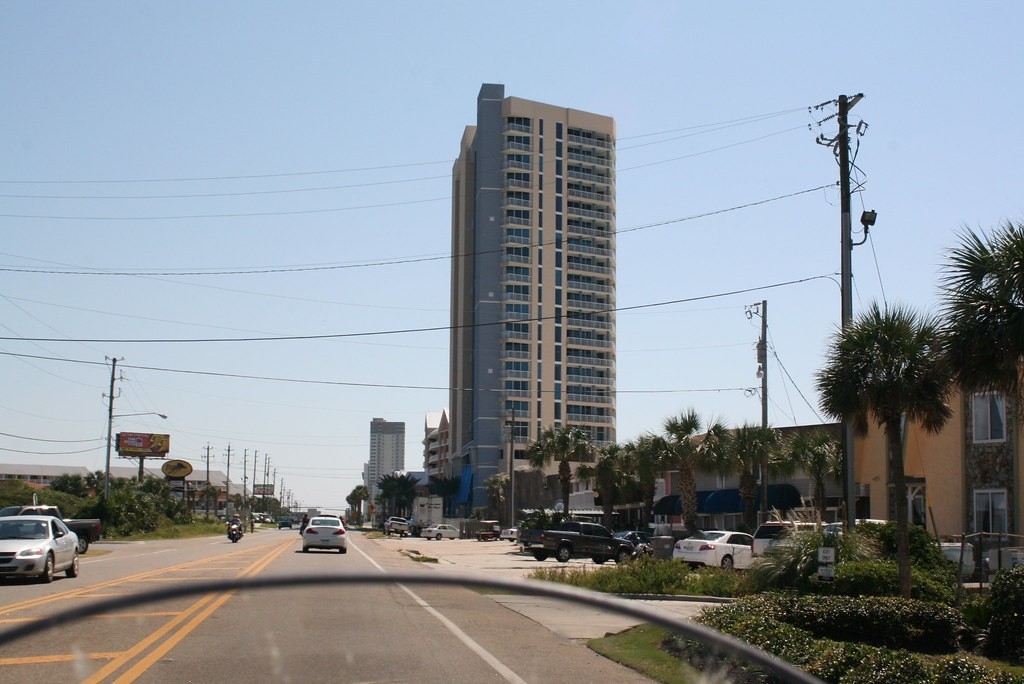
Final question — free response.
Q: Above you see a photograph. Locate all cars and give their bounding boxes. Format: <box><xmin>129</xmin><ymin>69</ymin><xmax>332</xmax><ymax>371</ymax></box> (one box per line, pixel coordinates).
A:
<box><xmin>419</xmin><ymin>523</ymin><xmax>466</xmax><ymax>541</ymax></box>
<box><xmin>615</xmin><ymin>530</ymin><xmax>653</xmax><ymax>548</ymax></box>
<box><xmin>499</xmin><ymin>525</ymin><xmax>522</xmax><ymax>542</ymax></box>
<box><xmin>302</xmin><ymin>516</ymin><xmax>348</xmax><ymax>553</ymax></box>
<box><xmin>0</xmin><ymin>516</ymin><xmax>80</xmax><ymax>584</ymax></box>
<box><xmin>672</xmin><ymin>529</ymin><xmax>754</xmax><ymax>573</ymax></box>
<box><xmin>277</xmin><ymin>515</ymin><xmax>292</xmax><ymax>529</ymax></box>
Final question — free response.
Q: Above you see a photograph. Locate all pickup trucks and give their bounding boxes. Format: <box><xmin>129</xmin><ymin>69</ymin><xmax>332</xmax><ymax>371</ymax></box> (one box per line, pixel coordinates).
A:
<box><xmin>0</xmin><ymin>504</ymin><xmax>102</xmax><ymax>554</ymax></box>
<box><xmin>384</xmin><ymin>516</ymin><xmax>408</xmax><ymax>537</ymax></box>
<box><xmin>519</xmin><ymin>520</ymin><xmax>635</xmax><ymax>564</ymax></box>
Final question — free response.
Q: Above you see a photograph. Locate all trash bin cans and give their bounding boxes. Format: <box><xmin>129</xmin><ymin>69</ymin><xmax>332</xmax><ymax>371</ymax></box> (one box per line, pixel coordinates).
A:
<box><xmin>649</xmin><ymin>535</ymin><xmax>674</xmax><ymax>561</ymax></box>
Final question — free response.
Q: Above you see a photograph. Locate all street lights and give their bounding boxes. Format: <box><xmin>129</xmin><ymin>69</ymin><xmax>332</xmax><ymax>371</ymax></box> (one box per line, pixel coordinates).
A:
<box><xmin>754</xmin><ymin>363</ymin><xmax>769</xmax><ymax>522</ymax></box>
<box><xmin>102</xmin><ymin>412</ymin><xmax>168</xmax><ymax>540</ymax></box>
<box><xmin>840</xmin><ymin>210</ymin><xmax>878</xmax><ymax>537</ymax></box>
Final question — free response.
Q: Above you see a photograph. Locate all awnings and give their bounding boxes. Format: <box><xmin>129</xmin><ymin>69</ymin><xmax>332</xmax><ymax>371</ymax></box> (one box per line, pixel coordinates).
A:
<box><xmin>648</xmin><ymin>483</ymin><xmax>870</xmax><ymax>520</ymax></box>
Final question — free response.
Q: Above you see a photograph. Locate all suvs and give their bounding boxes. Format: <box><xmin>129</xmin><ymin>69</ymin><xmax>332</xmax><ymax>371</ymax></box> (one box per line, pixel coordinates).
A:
<box><xmin>750</xmin><ymin>520</ymin><xmax>828</xmax><ymax>571</ymax></box>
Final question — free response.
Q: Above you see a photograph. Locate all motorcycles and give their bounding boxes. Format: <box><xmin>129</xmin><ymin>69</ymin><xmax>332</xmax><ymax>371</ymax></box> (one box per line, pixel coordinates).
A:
<box><xmin>224</xmin><ymin>522</ymin><xmax>245</xmax><ymax>543</ymax></box>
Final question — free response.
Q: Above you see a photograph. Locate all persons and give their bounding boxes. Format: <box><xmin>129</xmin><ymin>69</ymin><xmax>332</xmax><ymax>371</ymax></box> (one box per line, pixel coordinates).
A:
<box><xmin>226</xmin><ymin>512</ymin><xmax>273</xmax><ymax>539</ymax></box>
<box><xmin>294</xmin><ymin>514</ymin><xmax>310</xmax><ymax>535</ymax></box>
<box><xmin>341</xmin><ymin>515</ymin><xmax>349</xmax><ymax>532</ymax></box>
<box><xmin>462</xmin><ymin>524</ymin><xmax>499</xmax><ymax>542</ymax></box>
<box><xmin>409</xmin><ymin>518</ymin><xmax>413</xmax><ymax>529</ymax></box>
